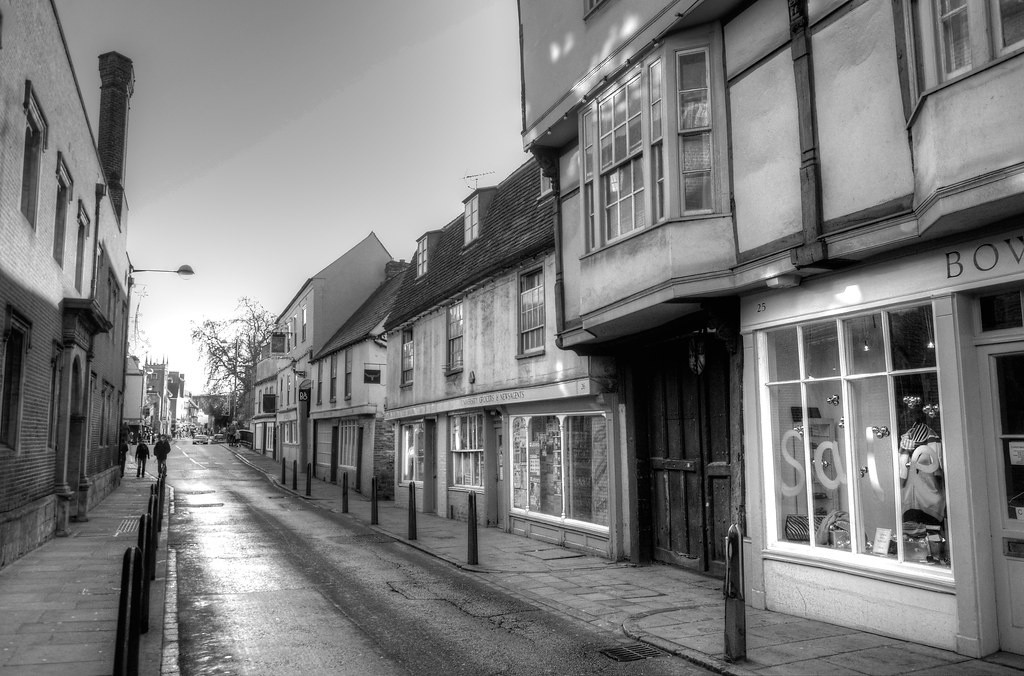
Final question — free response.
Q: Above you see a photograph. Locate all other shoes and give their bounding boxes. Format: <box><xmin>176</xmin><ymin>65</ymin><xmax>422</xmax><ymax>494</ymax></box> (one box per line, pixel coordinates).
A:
<box><xmin>165</xmin><ymin>474</ymin><xmax>168</xmax><ymax>476</ymax></box>
<box><xmin>158</xmin><ymin>473</ymin><xmax>161</xmax><ymax>478</ymax></box>
<box><xmin>136</xmin><ymin>475</ymin><xmax>139</xmax><ymax>477</ymax></box>
<box><xmin>142</xmin><ymin>475</ymin><xmax>144</xmax><ymax>477</ymax></box>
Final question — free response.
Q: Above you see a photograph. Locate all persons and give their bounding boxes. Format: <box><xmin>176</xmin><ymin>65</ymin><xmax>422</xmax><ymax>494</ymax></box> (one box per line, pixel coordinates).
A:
<box><xmin>227</xmin><ymin>426</ymin><xmax>241</xmax><ymax>448</ymax></box>
<box><xmin>121</xmin><ymin>439</ymin><xmax>128</xmax><ymax>478</ymax></box>
<box><xmin>135</xmin><ymin>439</ymin><xmax>150</xmax><ymax>478</ymax></box>
<box><xmin>899</xmin><ymin>404</ymin><xmax>946</xmax><ymax>527</ymax></box>
<box><xmin>128</xmin><ymin>427</ymin><xmax>208</xmax><ymax>442</ymax></box>
<box><xmin>154</xmin><ymin>435</ymin><xmax>171</xmax><ymax>478</ymax></box>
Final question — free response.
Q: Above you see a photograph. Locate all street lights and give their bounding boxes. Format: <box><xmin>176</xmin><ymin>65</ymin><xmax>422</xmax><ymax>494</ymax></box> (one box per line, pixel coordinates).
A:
<box><xmin>128</xmin><ymin>265</ymin><xmax>195</xmax><ymax>297</ymax></box>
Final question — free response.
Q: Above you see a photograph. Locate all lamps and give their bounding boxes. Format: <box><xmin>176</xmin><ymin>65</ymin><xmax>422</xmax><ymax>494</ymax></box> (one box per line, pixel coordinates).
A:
<box><xmin>130</xmin><ymin>265</ymin><xmax>195</xmax><ymax>275</ymax></box>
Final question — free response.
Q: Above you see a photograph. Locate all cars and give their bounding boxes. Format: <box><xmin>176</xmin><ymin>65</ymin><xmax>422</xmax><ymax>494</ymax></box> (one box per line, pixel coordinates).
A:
<box><xmin>193</xmin><ymin>435</ymin><xmax>208</xmax><ymax>445</ymax></box>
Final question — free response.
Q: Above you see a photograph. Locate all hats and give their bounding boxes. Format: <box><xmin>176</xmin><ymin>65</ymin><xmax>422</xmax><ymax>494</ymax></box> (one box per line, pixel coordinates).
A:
<box><xmin>138</xmin><ymin>438</ymin><xmax>145</xmax><ymax>442</ymax></box>
<box><xmin>159</xmin><ymin>434</ymin><xmax>167</xmax><ymax>437</ymax></box>
